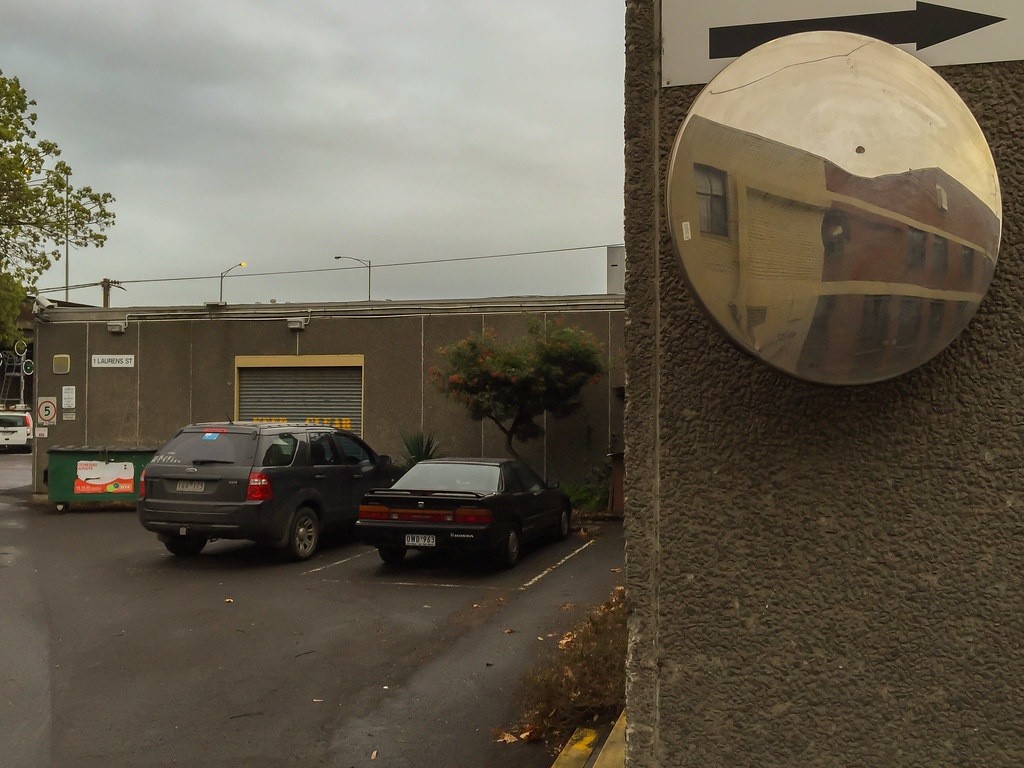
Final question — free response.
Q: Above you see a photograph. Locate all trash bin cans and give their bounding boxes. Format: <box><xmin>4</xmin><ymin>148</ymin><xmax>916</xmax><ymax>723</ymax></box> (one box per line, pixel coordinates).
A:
<box><xmin>45</xmin><ymin>444</ymin><xmax>159</xmax><ymax>514</ymax></box>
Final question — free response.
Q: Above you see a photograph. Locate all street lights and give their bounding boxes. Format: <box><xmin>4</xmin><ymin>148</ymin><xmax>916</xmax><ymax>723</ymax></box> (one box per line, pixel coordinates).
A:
<box><xmin>220</xmin><ymin>262</ymin><xmax>246</xmax><ymax>302</ymax></box>
<box><xmin>334</xmin><ymin>256</ymin><xmax>371</xmax><ymax>301</ymax></box>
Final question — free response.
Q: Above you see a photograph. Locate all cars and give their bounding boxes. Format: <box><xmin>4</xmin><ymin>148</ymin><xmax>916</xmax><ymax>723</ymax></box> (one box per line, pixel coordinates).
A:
<box><xmin>136</xmin><ymin>421</ymin><xmax>407</xmax><ymax>561</ymax></box>
<box><xmin>0</xmin><ymin>411</ymin><xmax>34</xmax><ymax>454</ymax></box>
<box><xmin>355</xmin><ymin>457</ymin><xmax>572</xmax><ymax>568</ymax></box>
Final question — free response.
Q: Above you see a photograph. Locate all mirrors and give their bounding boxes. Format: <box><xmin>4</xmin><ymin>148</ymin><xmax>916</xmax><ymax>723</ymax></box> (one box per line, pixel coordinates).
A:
<box><xmin>667</xmin><ymin>31</ymin><xmax>1002</xmax><ymax>386</ymax></box>
